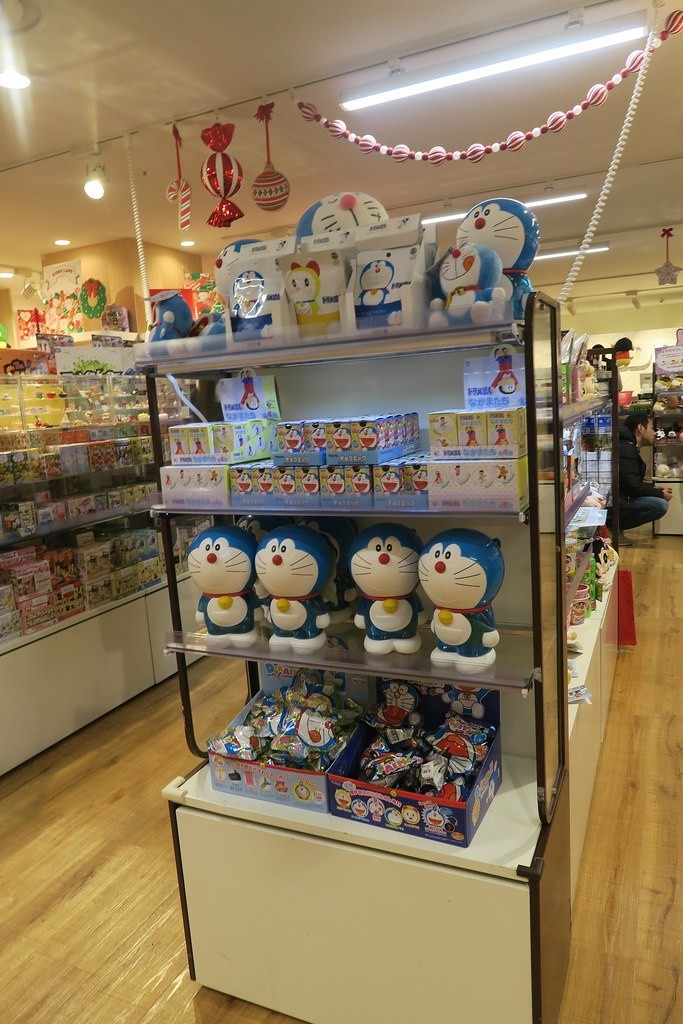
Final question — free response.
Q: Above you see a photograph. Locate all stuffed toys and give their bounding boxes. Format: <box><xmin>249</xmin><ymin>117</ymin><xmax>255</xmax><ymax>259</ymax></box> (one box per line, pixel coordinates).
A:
<box><xmin>429</xmin><ymin>245</ymin><xmax>506</xmax><ymax>328</ymax></box>
<box><xmin>614</xmin><ymin>338</ymin><xmax>632</xmax><ymax>367</ymax></box>
<box><xmin>581</xmin><ymin>539</ymin><xmax>615</xmax><ymax>579</ymax></box>
<box><xmin>583</xmin><ymin>364</ymin><xmax>598</xmax><ymax>395</ymax></box>
<box><xmin>652</xmin><ymin>376</ymin><xmax>683</xmax><ymax>478</ymax></box>
<box><xmin>296</xmin><ymin>190</ymin><xmax>388</xmax><ymax>237</ymax></box>
<box><xmin>146</xmin><ymin>294</ymin><xmax>227</xmax><ymax>361</ymax></box>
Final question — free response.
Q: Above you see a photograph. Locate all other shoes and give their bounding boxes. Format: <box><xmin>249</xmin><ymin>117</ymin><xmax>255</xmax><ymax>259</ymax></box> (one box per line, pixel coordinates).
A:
<box><xmin>610</xmin><ymin>530</ymin><xmax>633</xmax><ymax>546</ymax></box>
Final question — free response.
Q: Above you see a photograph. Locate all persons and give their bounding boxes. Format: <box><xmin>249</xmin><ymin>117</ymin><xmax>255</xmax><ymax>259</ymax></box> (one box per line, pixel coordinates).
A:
<box><xmin>605</xmin><ymin>414</ymin><xmax>673</xmax><ymax>545</ymax></box>
<box><xmin>589</xmin><ymin>344</ymin><xmax>623</xmax><ymax>392</ymax></box>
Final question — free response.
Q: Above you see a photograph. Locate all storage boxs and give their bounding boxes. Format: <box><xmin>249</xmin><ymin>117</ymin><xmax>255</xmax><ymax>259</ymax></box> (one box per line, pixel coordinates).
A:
<box><xmin>0</xmin><ymin>404</ymin><xmax>529</xmax><ymax>642</ymax></box>
<box><xmin>208</xmin><ymin>661</ymin><xmax>504</xmax><ymax>843</ymax></box>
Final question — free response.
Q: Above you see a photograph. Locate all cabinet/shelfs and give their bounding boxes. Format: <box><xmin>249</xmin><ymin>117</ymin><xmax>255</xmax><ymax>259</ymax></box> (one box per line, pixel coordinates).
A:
<box><xmin>0</xmin><ymin>371</ymin><xmax>220</xmax><ymax>776</ymax></box>
<box><xmin>646</xmin><ymin>353</ymin><xmax>683</xmax><ymax>537</ymax></box>
<box><xmin>158</xmin><ymin>290</ymin><xmax>564</xmax><ymax>1024</ymax></box>
<box><xmin>560</xmin><ymin>361</ymin><xmax>621</xmax><ymax>909</ymax></box>
